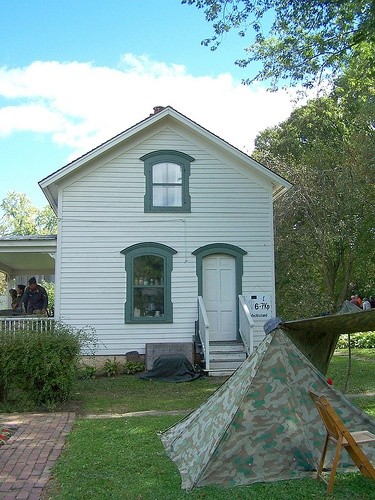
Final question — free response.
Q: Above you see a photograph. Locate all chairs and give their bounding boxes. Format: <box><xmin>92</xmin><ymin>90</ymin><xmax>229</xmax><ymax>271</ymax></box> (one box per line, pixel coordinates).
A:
<box><xmin>308</xmin><ymin>387</ymin><xmax>375</xmax><ymax>496</ymax></box>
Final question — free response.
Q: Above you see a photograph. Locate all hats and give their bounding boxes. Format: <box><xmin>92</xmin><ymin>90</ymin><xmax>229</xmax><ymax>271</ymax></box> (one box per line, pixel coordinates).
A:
<box><xmin>28</xmin><ymin>276</ymin><xmax>36</xmax><ymax>283</ymax></box>
<box><xmin>263</xmin><ymin>317</ymin><xmax>283</xmax><ymax>335</ymax></box>
<box><xmin>9</xmin><ymin>289</ymin><xmax>17</xmax><ymax>295</ymax></box>
<box><xmin>18</xmin><ymin>285</ymin><xmax>26</xmax><ymax>289</ymax></box>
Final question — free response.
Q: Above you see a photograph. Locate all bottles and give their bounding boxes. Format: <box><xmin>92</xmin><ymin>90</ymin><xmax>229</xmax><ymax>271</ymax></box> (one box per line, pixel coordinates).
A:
<box><xmin>135</xmin><ymin>277</ymin><xmax>163</xmax><ymax>286</ymax></box>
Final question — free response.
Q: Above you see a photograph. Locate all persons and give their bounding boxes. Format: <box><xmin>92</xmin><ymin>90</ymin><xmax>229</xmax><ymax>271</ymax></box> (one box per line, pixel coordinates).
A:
<box><xmin>9</xmin><ymin>278</ymin><xmax>48</xmax><ymax>314</ymax></box>
<box><xmin>350</xmin><ymin>294</ymin><xmax>375</xmax><ymax>310</ymax></box>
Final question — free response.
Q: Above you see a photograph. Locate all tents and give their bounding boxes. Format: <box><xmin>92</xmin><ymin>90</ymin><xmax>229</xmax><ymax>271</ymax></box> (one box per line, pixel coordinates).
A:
<box><xmin>158</xmin><ymin>327</ymin><xmax>374</xmax><ymax>489</ymax></box>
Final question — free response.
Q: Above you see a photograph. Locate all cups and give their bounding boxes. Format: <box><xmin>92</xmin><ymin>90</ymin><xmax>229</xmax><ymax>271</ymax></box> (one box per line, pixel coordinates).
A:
<box><xmin>155</xmin><ymin>311</ymin><xmax>160</xmax><ymax>317</ymax></box>
